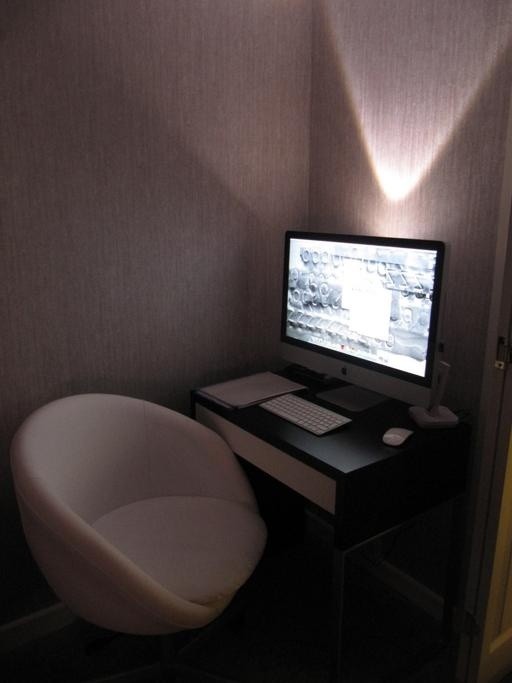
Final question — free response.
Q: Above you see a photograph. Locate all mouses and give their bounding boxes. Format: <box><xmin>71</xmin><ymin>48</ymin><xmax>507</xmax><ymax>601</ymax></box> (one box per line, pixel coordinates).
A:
<box><xmin>381</xmin><ymin>427</ymin><xmax>414</xmax><ymax>448</ymax></box>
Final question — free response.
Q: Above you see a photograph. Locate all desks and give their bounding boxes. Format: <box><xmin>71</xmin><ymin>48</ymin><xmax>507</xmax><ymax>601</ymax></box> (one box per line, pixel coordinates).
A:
<box><xmin>191</xmin><ymin>360</ymin><xmax>475</xmax><ymax>683</ymax></box>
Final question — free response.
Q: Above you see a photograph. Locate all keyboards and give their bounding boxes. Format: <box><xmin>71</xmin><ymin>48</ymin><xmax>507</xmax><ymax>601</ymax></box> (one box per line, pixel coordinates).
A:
<box><xmin>257</xmin><ymin>393</ymin><xmax>353</xmax><ymax>437</ymax></box>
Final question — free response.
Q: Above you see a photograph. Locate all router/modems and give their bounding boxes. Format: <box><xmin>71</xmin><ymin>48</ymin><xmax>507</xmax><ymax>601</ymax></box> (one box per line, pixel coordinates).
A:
<box><xmin>409</xmin><ymin>403</ymin><xmax>459</xmax><ymax>430</ymax></box>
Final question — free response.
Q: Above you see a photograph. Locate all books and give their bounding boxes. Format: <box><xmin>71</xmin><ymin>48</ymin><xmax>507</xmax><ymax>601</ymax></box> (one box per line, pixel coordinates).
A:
<box><xmin>195</xmin><ymin>369</ymin><xmax>307</xmax><ymax>409</ymax></box>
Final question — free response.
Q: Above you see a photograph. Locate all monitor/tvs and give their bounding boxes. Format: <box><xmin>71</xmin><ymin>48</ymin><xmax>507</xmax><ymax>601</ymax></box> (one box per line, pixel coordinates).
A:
<box><xmin>280</xmin><ymin>229</ymin><xmax>449</xmax><ymax>413</ymax></box>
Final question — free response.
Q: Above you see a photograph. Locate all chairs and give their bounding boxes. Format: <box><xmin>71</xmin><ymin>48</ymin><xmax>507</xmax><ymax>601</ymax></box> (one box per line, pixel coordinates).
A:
<box><xmin>11</xmin><ymin>393</ymin><xmax>268</xmax><ymax>683</ymax></box>
